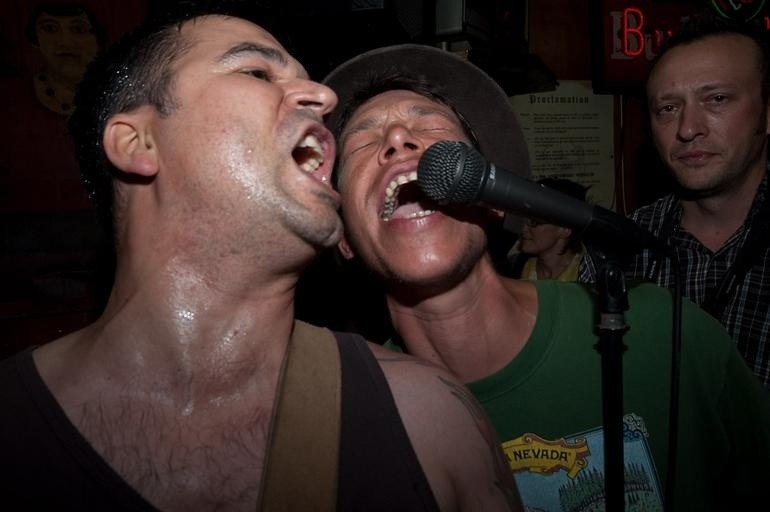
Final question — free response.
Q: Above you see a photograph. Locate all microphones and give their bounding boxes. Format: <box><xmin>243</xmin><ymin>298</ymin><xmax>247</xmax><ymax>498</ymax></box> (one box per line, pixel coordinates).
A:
<box><xmin>417</xmin><ymin>139</ymin><xmax>649</xmax><ymax>254</ymax></box>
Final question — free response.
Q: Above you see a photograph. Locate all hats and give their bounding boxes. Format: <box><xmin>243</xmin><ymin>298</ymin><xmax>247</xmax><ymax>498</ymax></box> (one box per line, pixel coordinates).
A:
<box><xmin>319</xmin><ymin>43</ymin><xmax>532</xmax><ymax>266</ymax></box>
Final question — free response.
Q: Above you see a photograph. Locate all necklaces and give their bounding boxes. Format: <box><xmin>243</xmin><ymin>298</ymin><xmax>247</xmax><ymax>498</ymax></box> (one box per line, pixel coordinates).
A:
<box><xmin>36</xmin><ymin>65</ymin><xmax>80</xmax><ymax>114</ymax></box>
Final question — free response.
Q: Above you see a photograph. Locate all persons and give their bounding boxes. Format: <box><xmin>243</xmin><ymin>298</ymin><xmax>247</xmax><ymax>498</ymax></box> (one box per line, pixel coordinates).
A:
<box><xmin>25</xmin><ymin>1</ymin><xmax>104</xmax><ymax>123</ymax></box>
<box><xmin>2</xmin><ymin>0</ymin><xmax>525</xmax><ymax>511</ymax></box>
<box><xmin>510</xmin><ymin>178</ymin><xmax>593</xmax><ymax>285</ymax></box>
<box><xmin>578</xmin><ymin>12</ymin><xmax>769</xmax><ymax>398</ymax></box>
<box><xmin>310</xmin><ymin>43</ymin><xmax>769</xmax><ymax>512</ymax></box>
